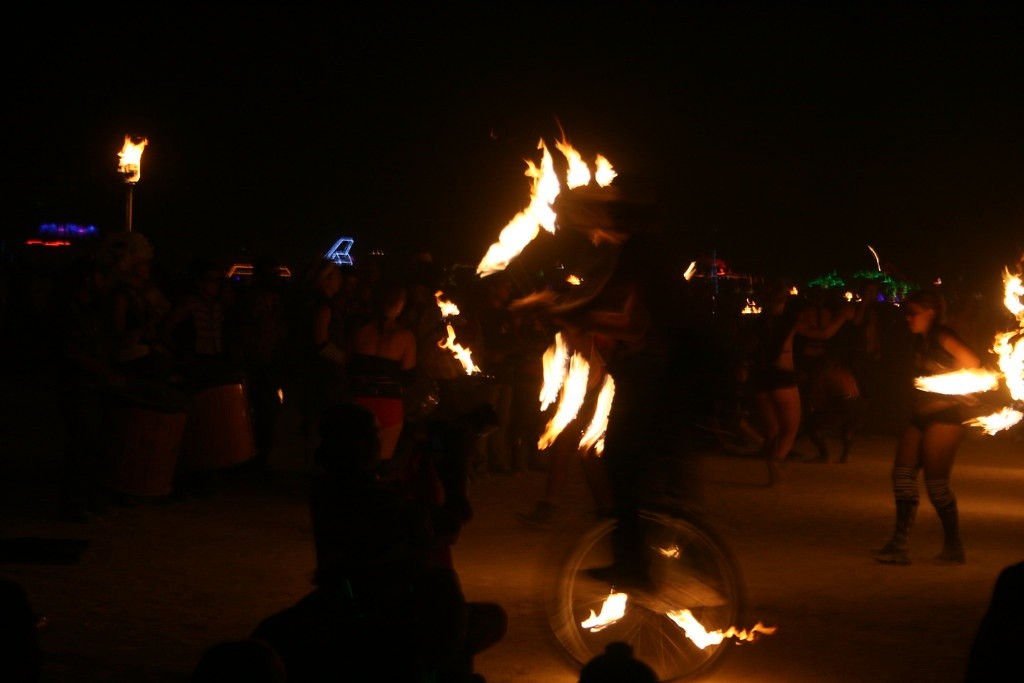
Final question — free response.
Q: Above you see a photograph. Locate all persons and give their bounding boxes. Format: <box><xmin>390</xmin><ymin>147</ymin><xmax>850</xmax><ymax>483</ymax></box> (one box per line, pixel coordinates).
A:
<box><xmin>573</xmin><ymin>642</ymin><xmax>665</xmax><ymax>682</ymax></box>
<box><xmin>1</xmin><ymin>574</ymin><xmax>55</xmax><ymax>682</ymax></box>
<box><xmin>294</xmin><ymin>395</ymin><xmax>461</xmax><ymax>682</ymax></box>
<box><xmin>188</xmin><ymin>636</ymin><xmax>288</xmax><ymax>681</ymax></box>
<box><xmin>866</xmin><ymin>290</ymin><xmax>969</xmax><ymax>565</ymax></box>
<box><xmin>44</xmin><ymin>224</ymin><xmax>885</xmax><ymax>581</ymax></box>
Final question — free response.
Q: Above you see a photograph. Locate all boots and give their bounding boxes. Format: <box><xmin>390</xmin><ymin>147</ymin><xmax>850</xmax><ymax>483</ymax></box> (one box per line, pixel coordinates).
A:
<box><xmin>838</xmin><ymin>426</ymin><xmax>854</xmax><ymax>463</ymax></box>
<box><xmin>870</xmin><ymin>500</ymin><xmax>919</xmax><ymax>565</ymax></box>
<box><xmin>932</xmin><ymin>500</ymin><xmax>964</xmax><ymax>564</ymax></box>
<box><xmin>806</xmin><ymin>430</ymin><xmax>830</xmax><ymax>464</ymax></box>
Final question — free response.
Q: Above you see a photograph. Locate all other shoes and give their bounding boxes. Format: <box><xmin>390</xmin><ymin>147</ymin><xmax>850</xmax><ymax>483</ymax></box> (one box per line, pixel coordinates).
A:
<box><xmin>513</xmin><ymin>501</ymin><xmax>561</xmax><ymax>530</ymax></box>
<box><xmin>766</xmin><ymin>458</ymin><xmax>801</xmax><ymax>488</ymax></box>
<box><xmin>578</xmin><ymin>513</ymin><xmax>657</xmax><ymax>593</ymax></box>
<box><xmin>489</xmin><ymin>463</ymin><xmax>511</xmax><ymax>474</ymax></box>
